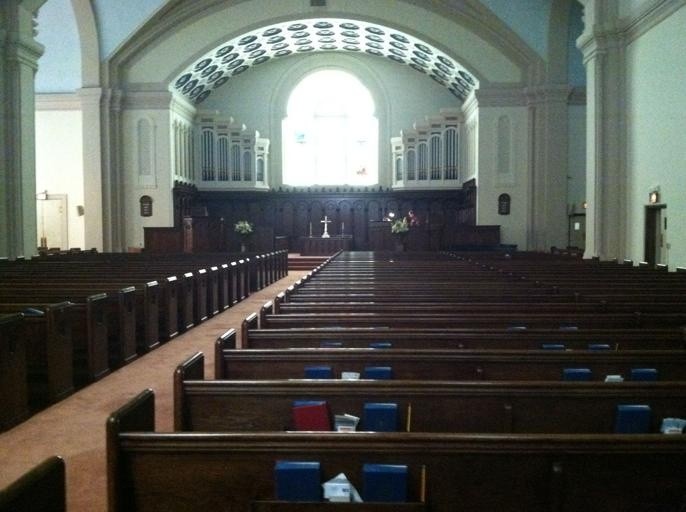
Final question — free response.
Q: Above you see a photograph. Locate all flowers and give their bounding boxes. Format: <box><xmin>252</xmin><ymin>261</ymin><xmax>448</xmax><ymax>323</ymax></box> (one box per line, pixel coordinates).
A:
<box><xmin>234</xmin><ymin>220</ymin><xmax>255</xmax><ymax>234</ymax></box>
<box><xmin>391</xmin><ymin>216</ymin><xmax>409</xmax><ymax>233</ymax></box>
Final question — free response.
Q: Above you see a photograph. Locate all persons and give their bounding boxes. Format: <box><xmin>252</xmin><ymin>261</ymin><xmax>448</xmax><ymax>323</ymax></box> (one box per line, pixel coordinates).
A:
<box><xmin>408</xmin><ymin>209</ymin><xmax>419</xmax><ymax>226</ymax></box>
